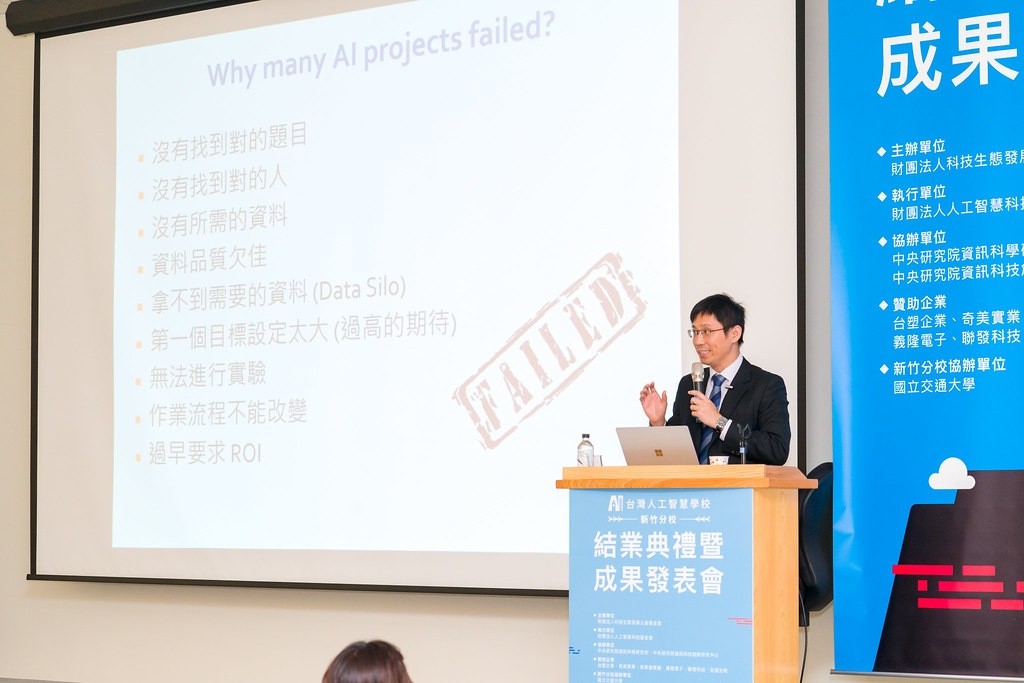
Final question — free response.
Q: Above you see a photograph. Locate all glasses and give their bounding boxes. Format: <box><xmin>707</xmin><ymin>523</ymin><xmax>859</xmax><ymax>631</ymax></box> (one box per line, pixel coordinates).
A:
<box><xmin>688</xmin><ymin>326</ymin><xmax>733</xmax><ymax>338</ymax></box>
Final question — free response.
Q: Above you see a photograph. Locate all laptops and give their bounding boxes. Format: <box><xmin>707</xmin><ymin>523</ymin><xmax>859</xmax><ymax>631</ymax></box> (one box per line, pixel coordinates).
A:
<box><xmin>616</xmin><ymin>425</ymin><xmax>700</xmax><ymax>465</ymax></box>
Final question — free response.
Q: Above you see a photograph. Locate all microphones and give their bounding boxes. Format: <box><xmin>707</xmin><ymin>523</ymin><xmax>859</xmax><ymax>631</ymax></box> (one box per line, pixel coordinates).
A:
<box><xmin>691</xmin><ymin>362</ymin><xmax>704</xmax><ymax>423</ymax></box>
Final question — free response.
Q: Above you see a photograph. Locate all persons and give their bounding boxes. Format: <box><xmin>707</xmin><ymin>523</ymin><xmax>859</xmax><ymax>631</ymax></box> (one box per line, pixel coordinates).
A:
<box><xmin>322</xmin><ymin>639</ymin><xmax>413</xmax><ymax>683</ymax></box>
<box><xmin>639</xmin><ymin>293</ymin><xmax>792</xmax><ymax>465</ymax></box>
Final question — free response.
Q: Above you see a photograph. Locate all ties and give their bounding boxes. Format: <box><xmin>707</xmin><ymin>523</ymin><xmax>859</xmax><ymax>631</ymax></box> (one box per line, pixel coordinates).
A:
<box><xmin>697</xmin><ymin>375</ymin><xmax>725</xmax><ymax>465</ymax></box>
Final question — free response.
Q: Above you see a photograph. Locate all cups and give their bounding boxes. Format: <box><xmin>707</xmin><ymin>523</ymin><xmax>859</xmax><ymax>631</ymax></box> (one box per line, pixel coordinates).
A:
<box><xmin>708</xmin><ymin>455</ymin><xmax>730</xmax><ymax>466</ymax></box>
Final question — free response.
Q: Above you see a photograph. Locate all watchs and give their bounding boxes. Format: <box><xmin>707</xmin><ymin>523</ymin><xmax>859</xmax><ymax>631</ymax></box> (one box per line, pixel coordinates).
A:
<box><xmin>716</xmin><ymin>416</ymin><xmax>728</xmax><ymax>434</ymax></box>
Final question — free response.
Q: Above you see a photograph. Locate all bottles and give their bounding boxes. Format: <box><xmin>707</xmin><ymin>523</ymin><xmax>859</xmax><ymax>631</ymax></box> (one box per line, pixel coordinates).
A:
<box><xmin>576</xmin><ymin>433</ymin><xmax>594</xmax><ymax>467</ymax></box>
